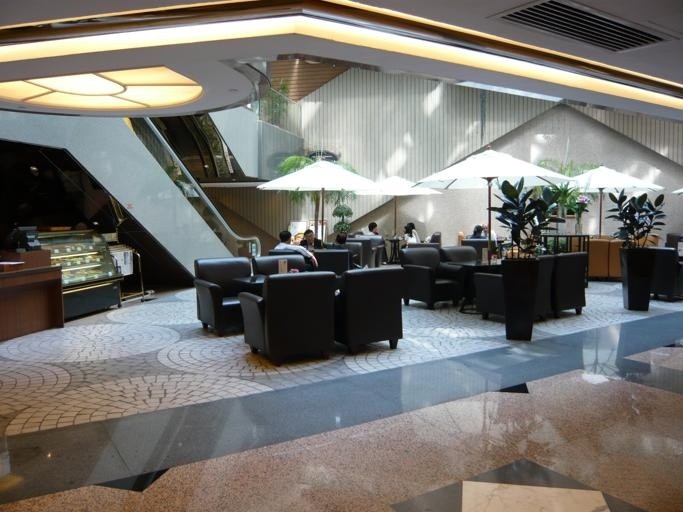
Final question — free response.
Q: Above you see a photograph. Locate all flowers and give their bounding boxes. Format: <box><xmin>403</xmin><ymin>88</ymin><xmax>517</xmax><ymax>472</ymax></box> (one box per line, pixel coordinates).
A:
<box><xmin>561</xmin><ymin>180</ymin><xmax>591</xmax><ymax>224</ymax></box>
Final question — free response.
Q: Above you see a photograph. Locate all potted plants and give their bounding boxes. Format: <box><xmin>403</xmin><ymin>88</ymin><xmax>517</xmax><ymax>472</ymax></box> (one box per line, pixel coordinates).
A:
<box><xmin>484</xmin><ymin>176</ymin><xmax>565</xmax><ymax>342</ymax></box>
<box><xmin>604</xmin><ymin>187</ymin><xmax>667</xmax><ymax>311</ymax></box>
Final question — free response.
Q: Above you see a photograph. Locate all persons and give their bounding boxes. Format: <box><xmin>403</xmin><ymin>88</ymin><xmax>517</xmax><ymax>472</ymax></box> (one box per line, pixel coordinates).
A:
<box><xmin>406</xmin><ymin>222</ymin><xmax>421</xmax><ymax>244</ymax></box>
<box><xmin>330</xmin><ymin>232</ymin><xmax>350</xmax><ymax>249</ymax></box>
<box><xmin>479</xmin><ymin>222</ymin><xmax>497</xmax><ymax>247</ymax></box>
<box><xmin>271</xmin><ymin>231</ymin><xmax>318</xmax><ymax>269</ymax></box>
<box><xmin>297</xmin><ymin>229</ymin><xmax>323</xmax><ymax>248</ymax></box>
<box><xmin>364</xmin><ymin>222</ymin><xmax>388</xmax><ymax>265</ymax></box>
<box><xmin>401</xmin><ymin>225</ymin><xmax>416</xmax><ymax>249</ymax></box>
<box><xmin>468</xmin><ymin>225</ymin><xmax>486</xmax><ymax>240</ymax></box>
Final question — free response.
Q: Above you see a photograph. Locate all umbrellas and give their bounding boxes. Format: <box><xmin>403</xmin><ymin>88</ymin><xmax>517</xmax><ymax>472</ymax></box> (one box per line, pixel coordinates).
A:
<box><xmin>254</xmin><ymin>159</ymin><xmax>384</xmax><ymax>249</ymax></box>
<box><xmin>355</xmin><ymin>175</ymin><xmax>442</xmax><ymax>236</ymax></box>
<box><xmin>545</xmin><ymin>166</ymin><xmax>664</xmax><ymax>239</ymax></box>
<box><xmin>409</xmin><ymin>145</ymin><xmax>570</xmax><ymax>259</ymax></box>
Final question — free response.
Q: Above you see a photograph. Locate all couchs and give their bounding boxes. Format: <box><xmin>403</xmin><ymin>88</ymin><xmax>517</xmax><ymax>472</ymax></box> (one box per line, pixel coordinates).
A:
<box><xmin>470</xmin><ymin>256</ymin><xmax>553</xmax><ymax>323</ymax></box>
<box><xmin>334</xmin><ymin>267</ymin><xmax>405</xmax><ymax>355</ymax></box>
<box><xmin>270</xmin><ymin>223</ymin><xmax>504</xmax><ymax>274</ymax></box>
<box><xmin>639</xmin><ymin>232</ymin><xmax>683</xmax><ymax>303</ymax></box>
<box><xmin>252</xmin><ymin>254</ymin><xmax>305</xmax><ymax>274</ymax></box>
<box><xmin>398</xmin><ymin>247</ymin><xmax>465</xmax><ymax>309</ymax></box>
<box><xmin>237</xmin><ymin>270</ymin><xmax>336</xmax><ymax>367</ymax></box>
<box><xmin>552</xmin><ymin>252</ymin><xmax>588</xmax><ymax>318</ymax></box>
<box><xmin>192</xmin><ymin>256</ymin><xmax>252</xmax><ymax>337</ymax></box>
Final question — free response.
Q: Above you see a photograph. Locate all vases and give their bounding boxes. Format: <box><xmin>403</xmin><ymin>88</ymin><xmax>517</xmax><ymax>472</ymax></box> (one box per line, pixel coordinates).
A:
<box><xmin>574</xmin><ymin>211</ymin><xmax>582</xmax><ymax>235</ymax></box>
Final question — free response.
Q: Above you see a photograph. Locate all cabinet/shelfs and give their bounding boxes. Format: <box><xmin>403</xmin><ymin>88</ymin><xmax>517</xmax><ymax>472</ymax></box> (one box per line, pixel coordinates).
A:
<box><xmin>18</xmin><ymin>226</ymin><xmax>124</xmax><ymax>323</ymax></box>
<box><xmin>539</xmin><ymin>233</ymin><xmax>590</xmax><ymax>289</ymax></box>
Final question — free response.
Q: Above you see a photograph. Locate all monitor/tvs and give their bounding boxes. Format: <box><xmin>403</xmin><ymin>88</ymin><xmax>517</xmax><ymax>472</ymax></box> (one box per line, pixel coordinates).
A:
<box><xmin>677</xmin><ymin>241</ymin><xmax>683</xmax><ymax>257</ymax></box>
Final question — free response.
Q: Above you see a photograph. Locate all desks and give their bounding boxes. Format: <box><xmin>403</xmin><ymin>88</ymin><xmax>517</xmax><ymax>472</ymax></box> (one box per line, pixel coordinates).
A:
<box><xmin>447</xmin><ymin>260</ymin><xmax>502</xmax><ymax>315</ymax></box>
